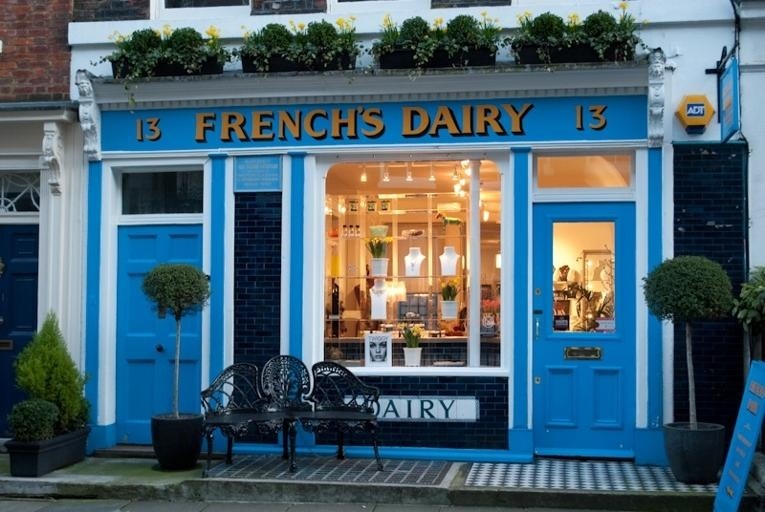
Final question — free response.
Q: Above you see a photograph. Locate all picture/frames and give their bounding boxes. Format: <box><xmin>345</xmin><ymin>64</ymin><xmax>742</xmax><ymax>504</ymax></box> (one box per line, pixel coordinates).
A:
<box><xmin>364</xmin><ymin>334</ymin><xmax>393</xmax><ymax>367</ymax></box>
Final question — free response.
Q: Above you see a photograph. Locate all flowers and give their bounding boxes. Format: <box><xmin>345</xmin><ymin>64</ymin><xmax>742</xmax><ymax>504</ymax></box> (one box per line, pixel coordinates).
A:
<box><xmin>362</xmin><ymin>236</ymin><xmax>394</xmax><ymax>257</ymax></box>
<box><xmin>438</xmin><ymin>275</ymin><xmax>463</xmax><ymax>301</ymax></box>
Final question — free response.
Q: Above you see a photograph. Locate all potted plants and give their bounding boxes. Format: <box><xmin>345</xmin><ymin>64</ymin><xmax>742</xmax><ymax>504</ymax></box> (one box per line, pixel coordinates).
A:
<box><xmin>401</xmin><ymin>327</ymin><xmax>423</xmax><ymax>367</ymax></box>
<box><xmin>140</xmin><ymin>262</ymin><xmax>214</xmax><ymax>471</ymax></box>
<box><xmin>639</xmin><ymin>255</ymin><xmax>734</xmax><ymax>485</ymax></box>
<box><xmin>4</xmin><ymin>308</ymin><xmax>94</xmax><ymax>478</ymax></box>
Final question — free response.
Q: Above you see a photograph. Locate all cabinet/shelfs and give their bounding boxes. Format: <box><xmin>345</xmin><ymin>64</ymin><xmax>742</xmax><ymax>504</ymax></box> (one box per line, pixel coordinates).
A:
<box><xmin>323</xmin><ymin>190</ymin><xmax>471</xmax><ymax>338</ymax></box>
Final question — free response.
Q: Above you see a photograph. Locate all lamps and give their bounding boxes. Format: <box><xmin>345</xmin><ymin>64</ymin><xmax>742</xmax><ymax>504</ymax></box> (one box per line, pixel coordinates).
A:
<box><xmin>359</xmin><ymin>161</ymin><xmax>459</xmax><ymax>183</ymax></box>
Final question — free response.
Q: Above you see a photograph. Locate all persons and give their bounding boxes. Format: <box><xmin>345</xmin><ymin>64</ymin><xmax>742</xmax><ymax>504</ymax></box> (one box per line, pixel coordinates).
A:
<box><xmin>366</xmin><ymin>335</ymin><xmax>387</xmax><ymax>362</ymax></box>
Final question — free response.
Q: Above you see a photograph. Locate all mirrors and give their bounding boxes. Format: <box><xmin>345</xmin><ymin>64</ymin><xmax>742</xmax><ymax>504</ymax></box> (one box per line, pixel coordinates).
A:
<box><xmin>582</xmin><ymin>249</ymin><xmax>614</xmax><ymax>331</ymax></box>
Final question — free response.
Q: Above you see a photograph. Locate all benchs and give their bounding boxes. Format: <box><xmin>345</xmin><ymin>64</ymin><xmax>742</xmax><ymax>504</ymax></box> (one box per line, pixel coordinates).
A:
<box><xmin>200</xmin><ymin>355</ymin><xmax>385</xmax><ymax>472</ymax></box>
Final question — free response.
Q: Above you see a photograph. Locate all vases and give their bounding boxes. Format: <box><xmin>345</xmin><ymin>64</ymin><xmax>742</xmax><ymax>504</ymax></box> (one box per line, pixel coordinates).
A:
<box><xmin>371</xmin><ymin>258</ymin><xmax>389</xmax><ymax>277</ymax></box>
<box><xmin>440</xmin><ymin>301</ymin><xmax>458</xmax><ymax>320</ymax></box>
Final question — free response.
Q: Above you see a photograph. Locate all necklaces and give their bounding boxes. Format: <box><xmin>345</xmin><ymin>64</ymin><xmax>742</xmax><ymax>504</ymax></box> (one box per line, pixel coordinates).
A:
<box><xmin>406</xmin><ymin>254</ymin><xmax>418</xmax><ymax>274</ymax></box>
<box><xmin>369</xmin><ymin>286</ymin><xmax>387</xmax><ymax>298</ymax></box>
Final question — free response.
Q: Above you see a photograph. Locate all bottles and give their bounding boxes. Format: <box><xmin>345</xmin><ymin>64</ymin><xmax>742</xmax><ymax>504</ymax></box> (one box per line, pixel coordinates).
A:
<box><xmin>341</xmin><ymin>224</ymin><xmax>361</xmax><ymax>238</ymax></box>
